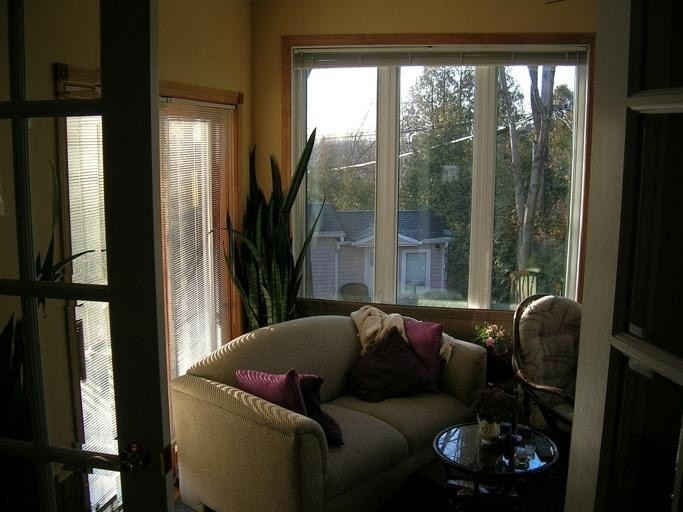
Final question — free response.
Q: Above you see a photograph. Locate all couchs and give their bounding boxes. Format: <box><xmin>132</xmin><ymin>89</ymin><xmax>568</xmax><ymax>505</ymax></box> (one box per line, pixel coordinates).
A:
<box><xmin>169</xmin><ymin>313</ymin><xmax>488</xmax><ymax>512</ymax></box>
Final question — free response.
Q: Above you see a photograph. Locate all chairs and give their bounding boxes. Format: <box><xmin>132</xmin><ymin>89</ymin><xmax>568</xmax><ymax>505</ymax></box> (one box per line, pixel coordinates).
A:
<box><xmin>511</xmin><ymin>293</ymin><xmax>618</xmax><ymax>450</ymax></box>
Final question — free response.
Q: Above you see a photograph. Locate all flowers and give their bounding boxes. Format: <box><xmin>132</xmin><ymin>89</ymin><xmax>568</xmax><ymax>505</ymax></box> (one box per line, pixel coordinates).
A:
<box><xmin>472</xmin><ymin>320</ymin><xmax>509</xmax><ymax>352</ymax></box>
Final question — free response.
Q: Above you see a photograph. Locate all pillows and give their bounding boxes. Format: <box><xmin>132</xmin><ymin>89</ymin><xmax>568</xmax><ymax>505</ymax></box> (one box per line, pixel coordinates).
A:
<box><xmin>346</xmin><ymin>326</ymin><xmax>441</xmax><ymax>403</ymax></box>
<box><xmin>298</xmin><ymin>373</ymin><xmax>344</xmax><ymax>446</ymax></box>
<box><xmin>401</xmin><ymin>316</ymin><xmax>443</xmax><ymax>385</ymax></box>
<box><xmin>234</xmin><ymin>367</ymin><xmax>308</xmax><ymax>417</ymax></box>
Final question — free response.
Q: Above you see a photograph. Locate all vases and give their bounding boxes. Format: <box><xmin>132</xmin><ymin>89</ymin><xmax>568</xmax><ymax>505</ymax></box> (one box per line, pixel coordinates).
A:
<box><xmin>487</xmin><ymin>346</ymin><xmax>508</xmax><ymax>368</ymax></box>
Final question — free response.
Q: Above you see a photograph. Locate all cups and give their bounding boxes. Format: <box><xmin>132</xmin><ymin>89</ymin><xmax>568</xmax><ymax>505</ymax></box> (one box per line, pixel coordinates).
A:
<box><xmin>514</xmin><ymin>444</ymin><xmax>538</xmax><ymax>462</ymax></box>
<box><xmin>513</xmin><ymin>462</ymin><xmax>529</xmax><ymax>470</ymax></box>
